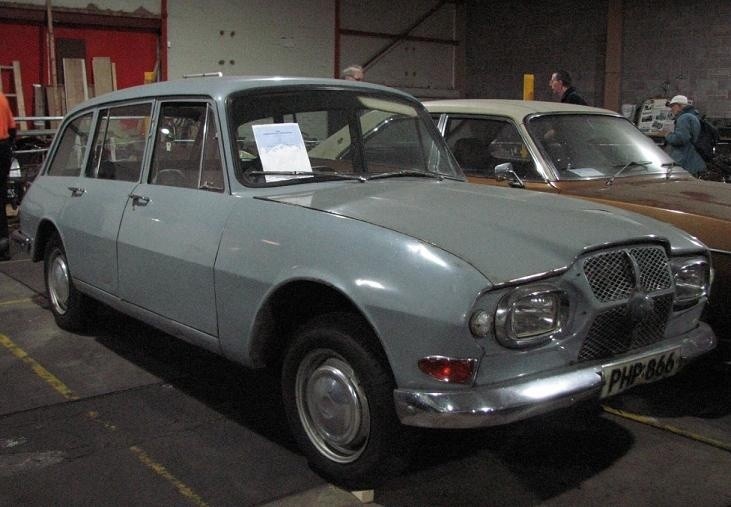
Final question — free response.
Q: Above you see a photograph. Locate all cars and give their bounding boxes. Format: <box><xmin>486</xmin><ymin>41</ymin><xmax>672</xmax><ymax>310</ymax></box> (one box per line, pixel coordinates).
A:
<box><xmin>13</xmin><ymin>71</ymin><xmax>717</xmax><ymax>483</ymax></box>
<box><xmin>308</xmin><ymin>98</ymin><xmax>730</xmax><ymax>361</ymax></box>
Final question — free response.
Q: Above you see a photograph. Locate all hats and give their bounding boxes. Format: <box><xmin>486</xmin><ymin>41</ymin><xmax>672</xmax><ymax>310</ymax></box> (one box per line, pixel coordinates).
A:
<box><xmin>670</xmin><ymin>95</ymin><xmax>687</xmax><ymax>104</ymax></box>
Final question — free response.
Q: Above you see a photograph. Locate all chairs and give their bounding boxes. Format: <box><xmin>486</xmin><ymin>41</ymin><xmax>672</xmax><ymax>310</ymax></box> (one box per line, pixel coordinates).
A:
<box><xmin>452</xmin><ymin>131</ymin><xmax>579</xmax><ymax>175</ymax></box>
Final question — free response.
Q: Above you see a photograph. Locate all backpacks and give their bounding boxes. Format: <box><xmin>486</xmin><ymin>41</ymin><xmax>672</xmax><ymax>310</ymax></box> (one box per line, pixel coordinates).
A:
<box><xmin>675</xmin><ymin>111</ymin><xmax>720</xmax><ymax>162</ymax></box>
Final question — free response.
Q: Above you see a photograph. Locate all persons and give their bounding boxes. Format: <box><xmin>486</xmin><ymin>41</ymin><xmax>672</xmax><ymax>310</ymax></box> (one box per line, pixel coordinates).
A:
<box><xmin>547</xmin><ymin>69</ymin><xmax>590</xmax><ymax>169</ymax></box>
<box><xmin>328</xmin><ymin>65</ymin><xmax>367</xmax><ymax>164</ymax></box>
<box><xmin>0</xmin><ymin>88</ymin><xmax>17</xmax><ymax>262</ymax></box>
<box><xmin>659</xmin><ymin>95</ymin><xmax>707</xmax><ymax>180</ymax></box>
